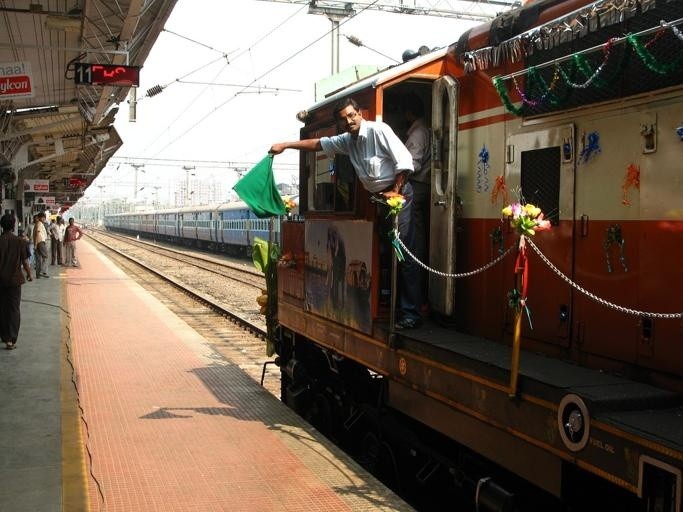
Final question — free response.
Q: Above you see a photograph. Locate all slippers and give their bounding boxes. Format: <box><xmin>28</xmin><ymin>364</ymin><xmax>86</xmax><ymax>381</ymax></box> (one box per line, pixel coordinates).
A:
<box><xmin>395</xmin><ymin>318</ymin><xmax>423</xmax><ymax>329</ymax></box>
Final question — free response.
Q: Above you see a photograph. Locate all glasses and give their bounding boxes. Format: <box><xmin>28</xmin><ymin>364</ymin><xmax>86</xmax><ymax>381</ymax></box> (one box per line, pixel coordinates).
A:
<box><xmin>340</xmin><ymin>112</ymin><xmax>357</xmax><ymax>123</ymax></box>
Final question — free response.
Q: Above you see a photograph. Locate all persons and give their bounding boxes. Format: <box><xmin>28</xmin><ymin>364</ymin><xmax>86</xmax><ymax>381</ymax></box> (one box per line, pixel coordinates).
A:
<box><xmin>267</xmin><ymin>96</ymin><xmax>431</xmax><ymax>330</ymax></box>
<box><xmin>0</xmin><ymin>214</ymin><xmax>34</xmax><ymax>350</ymax></box>
<box><xmin>24</xmin><ymin>213</ymin><xmax>82</xmax><ymax>279</ymax></box>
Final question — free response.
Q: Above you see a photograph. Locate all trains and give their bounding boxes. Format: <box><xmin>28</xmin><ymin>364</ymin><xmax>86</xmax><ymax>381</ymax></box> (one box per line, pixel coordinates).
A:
<box><xmin>104</xmin><ymin>195</ymin><xmax>305</xmax><ymax>258</ymax></box>
<box><xmin>257</xmin><ymin>0</ymin><xmax>683</xmax><ymax>512</ymax></box>
